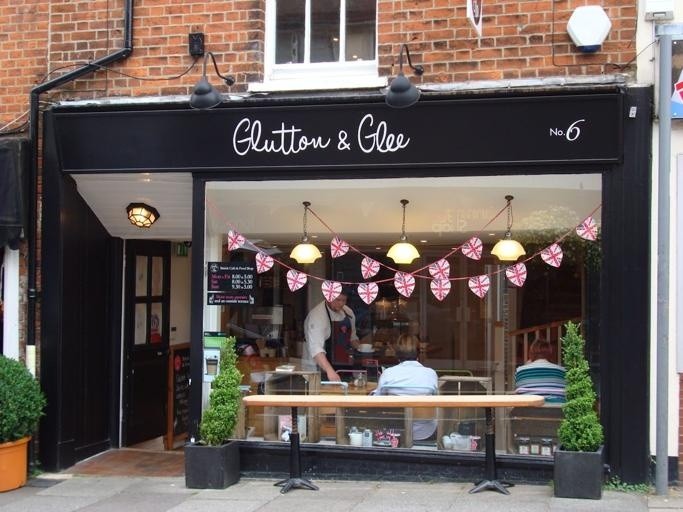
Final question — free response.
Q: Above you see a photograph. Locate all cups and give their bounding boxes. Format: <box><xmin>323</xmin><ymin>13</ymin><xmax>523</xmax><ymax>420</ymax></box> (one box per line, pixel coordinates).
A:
<box><xmin>358</xmin><ymin>343</ymin><xmax>372</xmax><ymax>351</ymax></box>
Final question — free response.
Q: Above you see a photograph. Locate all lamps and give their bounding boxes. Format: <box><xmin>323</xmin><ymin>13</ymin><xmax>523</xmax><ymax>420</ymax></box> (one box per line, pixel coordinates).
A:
<box><xmin>384</xmin><ymin>45</ymin><xmax>425</xmax><ymax>109</ymax></box>
<box><xmin>125</xmin><ymin>202</ymin><xmax>159</xmax><ymax>229</ymax></box>
<box><xmin>189</xmin><ymin>51</ymin><xmax>235</xmax><ymax>110</ymax></box>
<box><xmin>288</xmin><ymin>193</ymin><xmax>527</xmax><ymax>266</ymax></box>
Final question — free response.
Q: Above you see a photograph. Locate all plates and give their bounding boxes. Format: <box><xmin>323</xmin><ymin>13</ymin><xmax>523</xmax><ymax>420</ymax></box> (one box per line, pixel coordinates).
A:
<box><xmin>357</xmin><ymin>349</ymin><xmax>376</xmax><ymax>353</ymax></box>
<box><xmin>373</xmin><ymin>441</ymin><xmax>392</xmax><ymax>447</ymax></box>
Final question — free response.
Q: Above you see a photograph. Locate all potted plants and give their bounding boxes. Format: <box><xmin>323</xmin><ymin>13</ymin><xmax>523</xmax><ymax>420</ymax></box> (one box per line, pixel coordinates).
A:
<box><xmin>1</xmin><ymin>353</ymin><xmax>49</xmax><ymax>493</ymax></box>
<box><xmin>552</xmin><ymin>319</ymin><xmax>606</xmax><ymax>500</ymax></box>
<box><xmin>184</xmin><ymin>334</ymin><xmax>246</xmax><ymax>490</ymax></box>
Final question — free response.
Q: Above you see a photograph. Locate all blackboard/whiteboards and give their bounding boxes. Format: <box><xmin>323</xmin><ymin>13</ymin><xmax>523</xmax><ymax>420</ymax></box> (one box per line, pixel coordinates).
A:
<box><xmin>166</xmin><ymin>342</ymin><xmax>192</xmax><ymax>449</ymax></box>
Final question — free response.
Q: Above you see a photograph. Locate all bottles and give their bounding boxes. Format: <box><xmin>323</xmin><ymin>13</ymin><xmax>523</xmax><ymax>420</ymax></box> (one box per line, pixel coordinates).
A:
<box><xmin>362</xmin><ymin>429</ymin><xmax>372</xmax><ymax>446</ymax></box>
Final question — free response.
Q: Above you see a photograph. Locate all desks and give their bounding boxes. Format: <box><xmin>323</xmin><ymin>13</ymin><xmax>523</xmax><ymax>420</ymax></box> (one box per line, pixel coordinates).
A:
<box><xmin>243</xmin><ymin>395</ymin><xmax>545</xmax><ymax>495</ymax></box>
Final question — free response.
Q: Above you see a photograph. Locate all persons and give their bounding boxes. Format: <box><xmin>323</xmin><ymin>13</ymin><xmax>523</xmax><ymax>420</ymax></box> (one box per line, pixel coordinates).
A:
<box><xmin>301</xmin><ymin>286</ymin><xmax>361</xmax><ymax>384</ymax></box>
<box><xmin>367</xmin><ymin>334</ymin><xmax>439</xmax><ymax>442</ymax></box>
<box><xmin>510</xmin><ymin>336</ymin><xmax>569</xmax><ymax>445</ymax></box>
